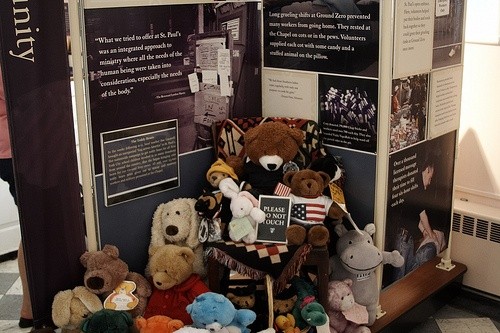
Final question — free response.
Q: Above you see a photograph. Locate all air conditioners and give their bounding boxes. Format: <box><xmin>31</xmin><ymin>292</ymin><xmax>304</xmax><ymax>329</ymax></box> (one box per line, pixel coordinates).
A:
<box><xmin>449</xmin><ymin>198</ymin><xmax>500</xmax><ymax>297</ymax></box>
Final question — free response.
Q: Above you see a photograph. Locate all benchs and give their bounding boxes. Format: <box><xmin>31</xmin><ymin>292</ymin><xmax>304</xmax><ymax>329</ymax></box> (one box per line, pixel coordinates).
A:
<box><xmin>367</xmin><ymin>256</ymin><xmax>469</xmax><ymax>333</ymax></box>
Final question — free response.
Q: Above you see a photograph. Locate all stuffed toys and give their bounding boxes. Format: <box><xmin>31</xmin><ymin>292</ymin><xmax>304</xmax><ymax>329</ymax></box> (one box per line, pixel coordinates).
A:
<box><xmin>51</xmin><ymin>120</ymin><xmax>404</xmax><ymax>333</ymax></box>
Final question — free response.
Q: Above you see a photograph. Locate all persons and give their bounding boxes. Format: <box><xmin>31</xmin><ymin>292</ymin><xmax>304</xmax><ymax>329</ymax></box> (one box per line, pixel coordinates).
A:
<box><xmin>396</xmin><ymin>156</ymin><xmax>445</xmax><ymax>278</ymax></box>
<box><xmin>449</xmin><ymin>0</ymin><xmax>462</xmax><ymax>57</ymax></box>
<box><xmin>392</xmin><ymin>74</ymin><xmax>426</xmax><ymax>141</ymax></box>
<box><xmin>0</xmin><ymin>63</ymin><xmax>35</xmax><ymax>329</ymax></box>
